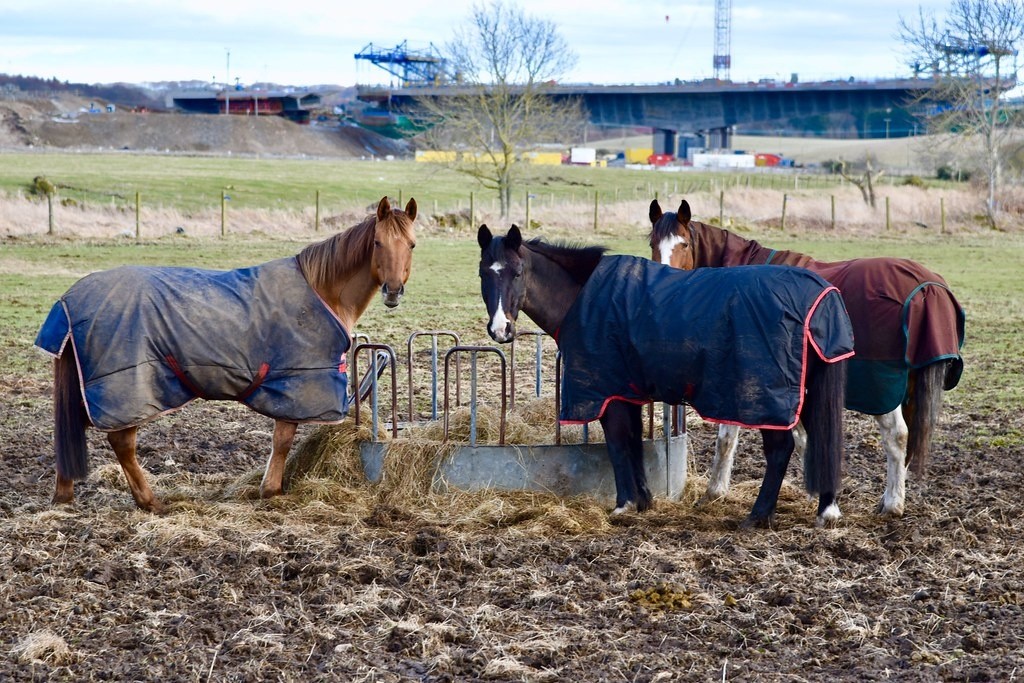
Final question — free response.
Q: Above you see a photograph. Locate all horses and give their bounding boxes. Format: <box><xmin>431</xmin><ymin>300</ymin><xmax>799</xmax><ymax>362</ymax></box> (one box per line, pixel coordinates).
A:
<box><xmin>648</xmin><ymin>197</ymin><xmax>968</xmax><ymax>515</ymax></box>
<box><xmin>31</xmin><ymin>196</ymin><xmax>419</xmax><ymax>512</ymax></box>
<box><xmin>475</xmin><ymin>222</ymin><xmax>857</xmax><ymax>535</ymax></box>
